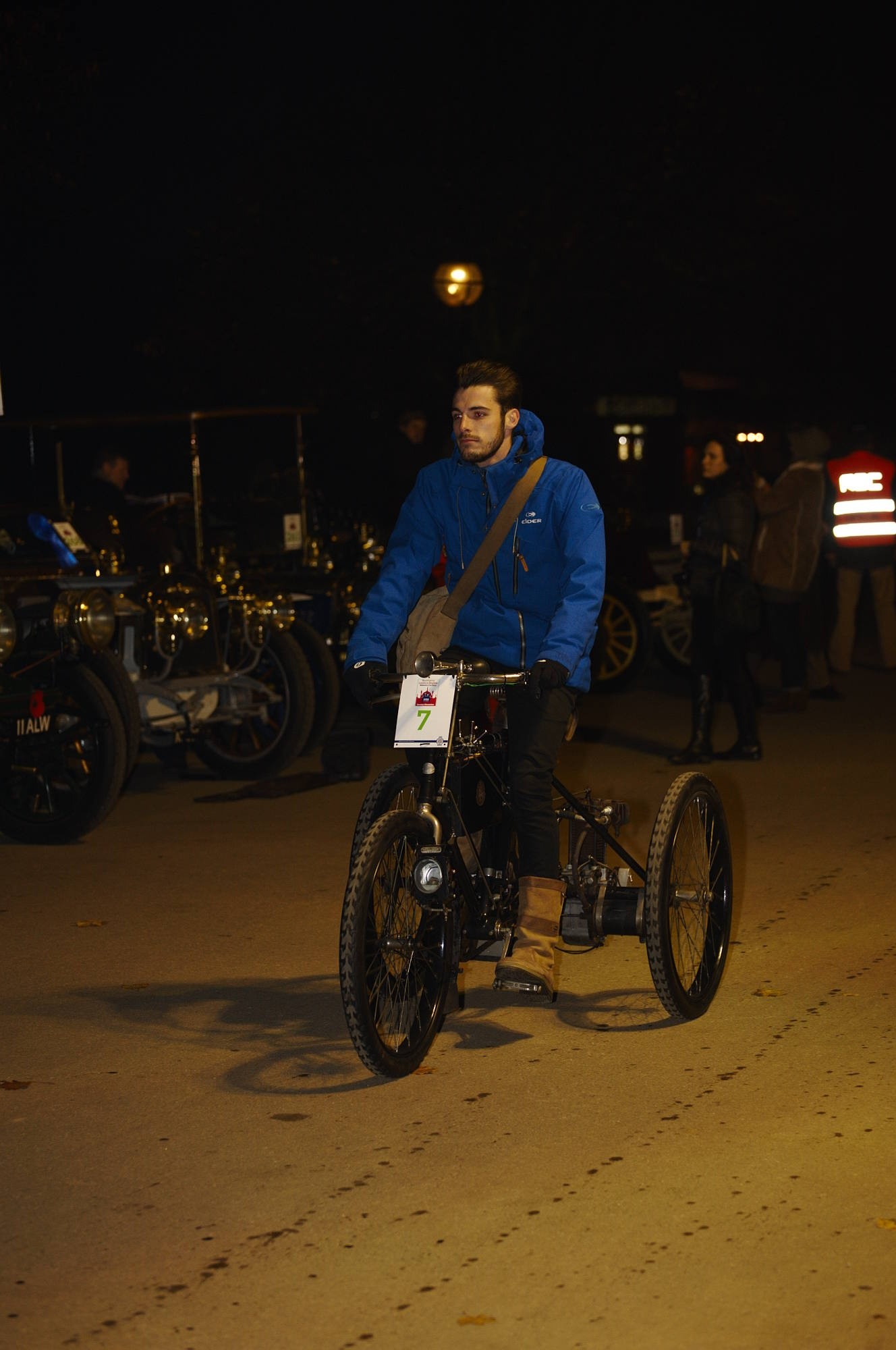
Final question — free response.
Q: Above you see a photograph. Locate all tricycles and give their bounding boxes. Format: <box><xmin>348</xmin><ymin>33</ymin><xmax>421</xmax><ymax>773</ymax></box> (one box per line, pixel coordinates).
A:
<box><xmin>335</xmin><ymin>652</ymin><xmax>733</xmax><ymax>1084</ymax></box>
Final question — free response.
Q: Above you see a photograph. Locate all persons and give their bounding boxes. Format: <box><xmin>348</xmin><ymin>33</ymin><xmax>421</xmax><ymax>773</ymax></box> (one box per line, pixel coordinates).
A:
<box><xmin>668</xmin><ymin>431</ymin><xmax>763</xmax><ymax>764</ymax></box>
<box><xmin>344</xmin><ymin>358</ymin><xmax>610</xmax><ymax>1009</ymax></box>
<box><xmin>67</xmin><ymin>448</ymin><xmax>193</xmax><ymax>565</ymax></box>
<box><xmin>741</xmin><ymin>423</ymin><xmax>830</xmax><ymax>708</ymax></box>
<box><xmin>829</xmin><ymin>425</ymin><xmax>896</xmax><ymax>675</ymax></box>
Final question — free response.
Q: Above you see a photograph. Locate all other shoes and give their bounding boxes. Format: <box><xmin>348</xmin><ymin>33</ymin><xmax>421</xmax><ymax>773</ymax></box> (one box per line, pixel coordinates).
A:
<box><xmin>808</xmin><ymin>684</ymin><xmax>832</xmax><ymax>699</ymax></box>
<box><xmin>757</xmin><ymin>686</ymin><xmax>808</xmax><ymax>714</ymax></box>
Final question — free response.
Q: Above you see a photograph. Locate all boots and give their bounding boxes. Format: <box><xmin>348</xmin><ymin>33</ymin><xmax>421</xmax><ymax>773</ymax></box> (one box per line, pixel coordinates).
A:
<box><xmin>712</xmin><ymin>680</ymin><xmax>762</xmax><ymax>760</ymax></box>
<box><xmin>495</xmin><ymin>875</ymin><xmax>567</xmax><ymax>1005</ymax></box>
<box><xmin>667</xmin><ymin>671</ymin><xmax>714</xmax><ymax>763</ymax></box>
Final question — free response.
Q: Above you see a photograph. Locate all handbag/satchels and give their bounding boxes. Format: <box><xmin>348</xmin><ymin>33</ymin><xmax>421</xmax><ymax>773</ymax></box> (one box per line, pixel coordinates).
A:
<box><xmin>395</xmin><ymin>585</ymin><xmax>457</xmax><ymax>692</ymax></box>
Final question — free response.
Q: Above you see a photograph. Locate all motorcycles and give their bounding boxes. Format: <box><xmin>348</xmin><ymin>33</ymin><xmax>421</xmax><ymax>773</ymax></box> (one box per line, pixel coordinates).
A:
<box><xmin>0</xmin><ymin>517</ymin><xmax>426</xmax><ymax>849</ymax></box>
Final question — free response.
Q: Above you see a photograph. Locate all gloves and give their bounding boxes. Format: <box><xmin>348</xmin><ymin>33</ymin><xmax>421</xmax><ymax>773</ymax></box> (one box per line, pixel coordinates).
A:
<box><xmin>342</xmin><ymin>660</ymin><xmax>389</xmax><ymax>707</ymax></box>
<box><xmin>527</xmin><ymin>659</ymin><xmax>568</xmax><ymax>700</ymax></box>
